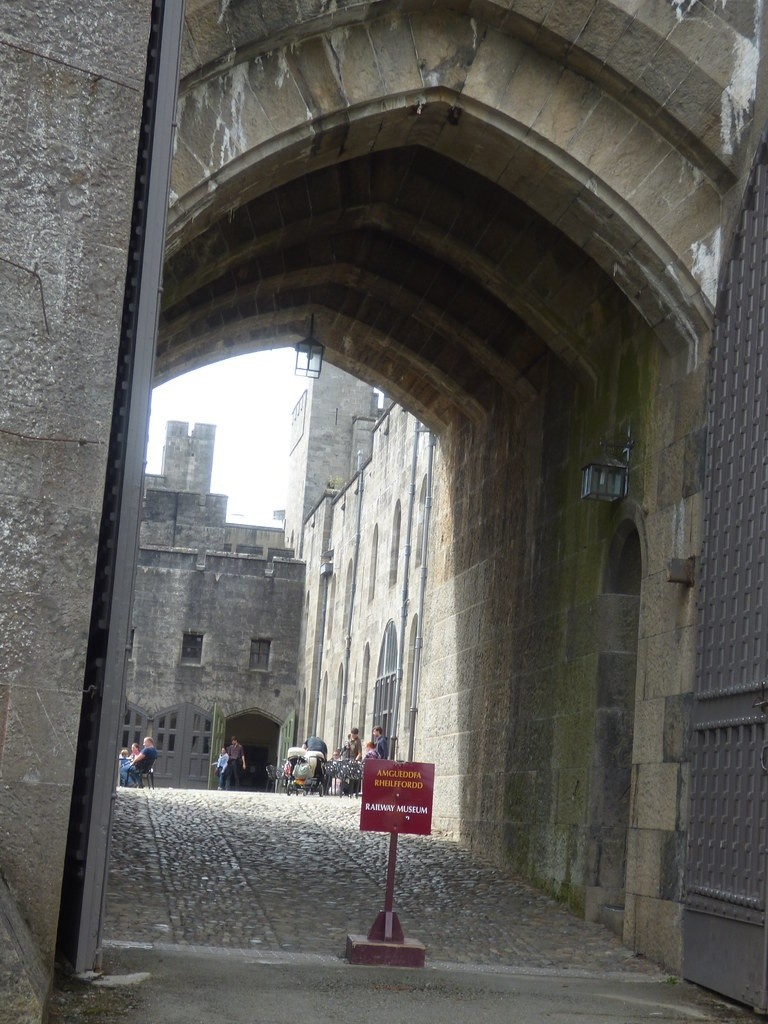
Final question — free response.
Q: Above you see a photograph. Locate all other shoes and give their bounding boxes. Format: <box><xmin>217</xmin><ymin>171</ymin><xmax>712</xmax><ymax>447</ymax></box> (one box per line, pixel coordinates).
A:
<box><xmin>217</xmin><ymin>787</ymin><xmax>224</xmax><ymax>790</ymax></box>
<box><xmin>138</xmin><ymin>784</ymin><xmax>145</xmax><ymax>788</ymax></box>
<box><xmin>132</xmin><ymin>783</ymin><xmax>139</xmax><ymax>788</ymax></box>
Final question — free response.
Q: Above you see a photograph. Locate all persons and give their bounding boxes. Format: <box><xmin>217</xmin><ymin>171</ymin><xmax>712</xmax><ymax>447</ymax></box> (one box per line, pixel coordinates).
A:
<box><xmin>211</xmin><ymin>724</ymin><xmax>388</xmax><ymax>796</ymax></box>
<box><xmin>119</xmin><ymin>736</ymin><xmax>157</xmax><ymax>788</ymax></box>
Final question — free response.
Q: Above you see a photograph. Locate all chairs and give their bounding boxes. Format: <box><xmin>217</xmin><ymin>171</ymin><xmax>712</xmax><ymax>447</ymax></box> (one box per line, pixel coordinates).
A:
<box><xmin>266</xmin><ymin>762</ymin><xmax>362</xmax><ymax>799</ymax></box>
<box><xmin>127</xmin><ymin>758</ymin><xmax>155</xmax><ymax>789</ymax></box>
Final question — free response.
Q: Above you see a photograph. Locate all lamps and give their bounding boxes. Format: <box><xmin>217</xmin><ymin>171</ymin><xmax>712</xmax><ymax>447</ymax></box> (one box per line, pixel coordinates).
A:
<box><xmin>294</xmin><ymin>314</ymin><xmax>325</xmax><ymax>379</ymax></box>
<box><xmin>581</xmin><ymin>433</ymin><xmax>634</xmax><ymax>503</ymax></box>
